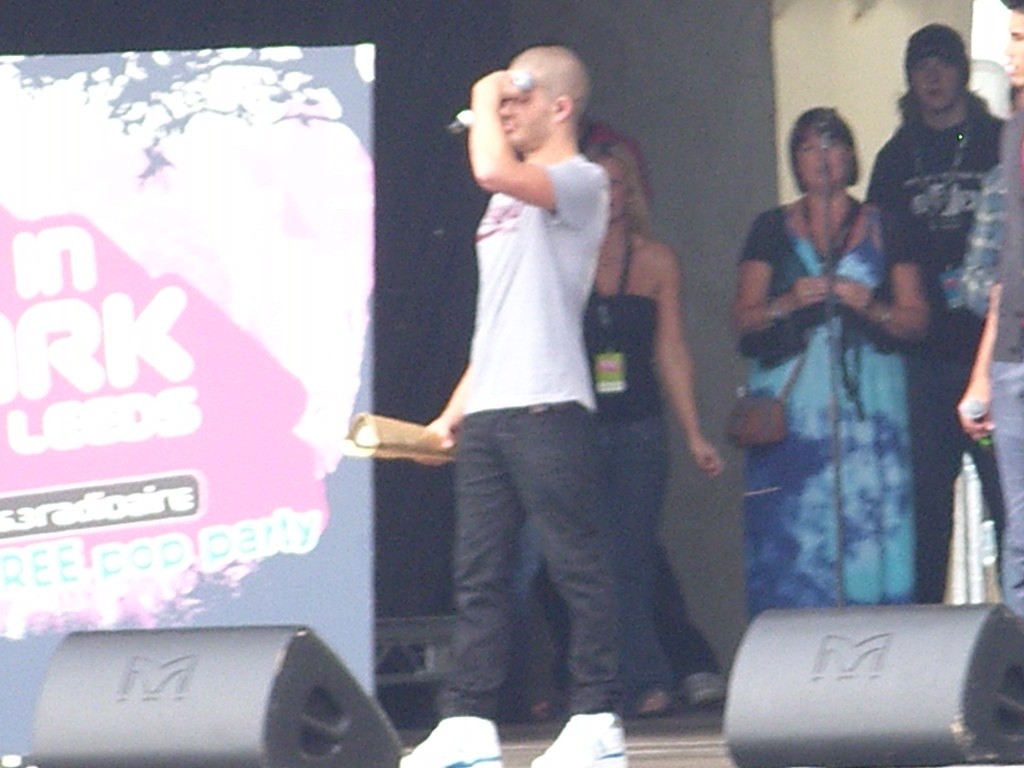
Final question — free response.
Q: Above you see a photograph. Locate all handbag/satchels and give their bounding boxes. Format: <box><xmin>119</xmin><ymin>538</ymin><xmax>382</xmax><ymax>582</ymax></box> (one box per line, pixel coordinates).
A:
<box><xmin>722</xmin><ymin>393</ymin><xmax>788</xmax><ymax>450</ymax></box>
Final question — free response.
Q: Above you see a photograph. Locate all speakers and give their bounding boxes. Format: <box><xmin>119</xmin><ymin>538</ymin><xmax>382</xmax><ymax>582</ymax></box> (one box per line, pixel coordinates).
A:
<box><xmin>721</xmin><ymin>603</ymin><xmax>1022</xmax><ymax>768</ymax></box>
<box><xmin>29</xmin><ymin>626</ymin><xmax>408</xmax><ymax>768</ymax></box>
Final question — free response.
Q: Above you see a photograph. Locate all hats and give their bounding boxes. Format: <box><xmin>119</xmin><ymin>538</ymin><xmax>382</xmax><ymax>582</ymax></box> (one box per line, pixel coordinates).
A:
<box><xmin>905</xmin><ymin>23</ymin><xmax>968</xmax><ymax>80</ymax></box>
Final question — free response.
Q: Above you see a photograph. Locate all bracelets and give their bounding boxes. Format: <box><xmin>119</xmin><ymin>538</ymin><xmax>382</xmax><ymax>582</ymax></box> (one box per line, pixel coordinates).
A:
<box><xmin>879</xmin><ymin>303</ymin><xmax>891</xmax><ymax>326</ymax></box>
<box><xmin>766</xmin><ymin>296</ymin><xmax>782</xmax><ymax>321</ymax></box>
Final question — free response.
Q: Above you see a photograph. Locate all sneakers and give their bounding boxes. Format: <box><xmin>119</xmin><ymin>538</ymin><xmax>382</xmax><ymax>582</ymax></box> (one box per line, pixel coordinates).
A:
<box><xmin>531</xmin><ymin>710</ymin><xmax>629</xmax><ymax>768</ymax></box>
<box><xmin>400</xmin><ymin>716</ymin><xmax>503</xmax><ymax>768</ymax></box>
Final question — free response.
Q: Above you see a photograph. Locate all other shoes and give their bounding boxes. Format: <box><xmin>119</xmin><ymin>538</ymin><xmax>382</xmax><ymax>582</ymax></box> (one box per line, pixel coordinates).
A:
<box><xmin>638</xmin><ymin>684</ymin><xmax>670</xmax><ymax>721</ymax></box>
<box><xmin>680</xmin><ymin>671</ymin><xmax>725</xmax><ymax>710</ymax></box>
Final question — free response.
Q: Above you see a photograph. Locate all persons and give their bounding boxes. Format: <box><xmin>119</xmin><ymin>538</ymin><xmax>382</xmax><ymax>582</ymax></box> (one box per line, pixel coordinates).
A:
<box><xmin>574</xmin><ymin>117</ymin><xmax>722</xmax><ymax>715</ymax></box>
<box><xmin>738</xmin><ymin>103</ymin><xmax>930</xmax><ymax>622</ymax></box>
<box><xmin>956</xmin><ymin>88</ymin><xmax>1023</xmax><ymax>311</ymax></box>
<box><xmin>401</xmin><ymin>44</ymin><xmax>630</xmax><ymax>768</ymax></box>
<box><xmin>960</xmin><ymin>1</ymin><xmax>1023</xmax><ymax>614</ymax></box>
<box><xmin>867</xmin><ymin>23</ymin><xmax>1007</xmax><ymax>604</ymax></box>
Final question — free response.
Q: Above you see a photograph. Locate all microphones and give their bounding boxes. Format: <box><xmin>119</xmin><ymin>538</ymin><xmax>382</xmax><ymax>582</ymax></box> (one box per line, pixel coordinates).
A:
<box><xmin>445</xmin><ymin>66</ymin><xmax>536</xmax><ymax>135</ymax></box>
<box><xmin>815</xmin><ymin>109</ymin><xmax>836</xmax><ymax>136</ymax></box>
